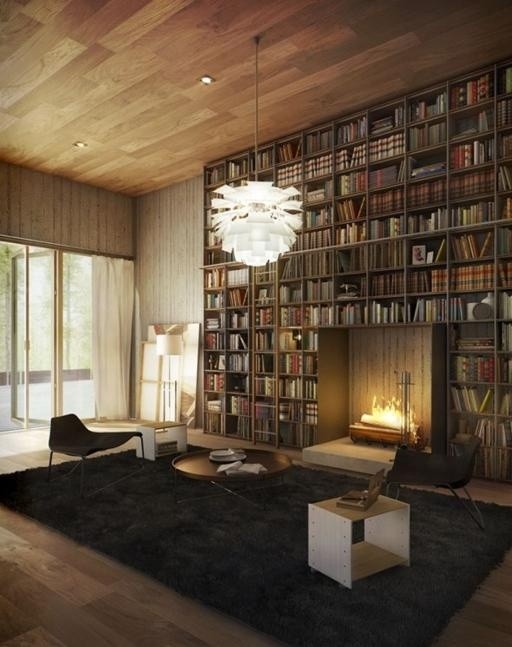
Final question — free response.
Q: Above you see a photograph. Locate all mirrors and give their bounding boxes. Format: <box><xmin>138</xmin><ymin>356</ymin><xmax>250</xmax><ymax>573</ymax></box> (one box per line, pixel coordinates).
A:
<box><xmin>136</xmin><ymin>320</ymin><xmax>202</xmax><ymax>430</ymax></box>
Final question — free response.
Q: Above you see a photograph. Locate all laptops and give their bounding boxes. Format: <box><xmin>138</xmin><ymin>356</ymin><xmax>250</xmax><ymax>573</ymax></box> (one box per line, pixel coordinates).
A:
<box><xmin>336</xmin><ymin>468</ymin><xmax>385</xmax><ymax>508</ymax></box>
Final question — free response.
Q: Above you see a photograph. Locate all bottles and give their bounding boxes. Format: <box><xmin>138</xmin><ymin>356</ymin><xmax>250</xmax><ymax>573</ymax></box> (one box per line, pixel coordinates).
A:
<box><xmin>208</xmin><ymin>354</ymin><xmax>216</xmax><ymax>368</ymax></box>
<box><xmin>480</xmin><ymin>292</ymin><xmax>493</xmax><ymax>320</ymax></box>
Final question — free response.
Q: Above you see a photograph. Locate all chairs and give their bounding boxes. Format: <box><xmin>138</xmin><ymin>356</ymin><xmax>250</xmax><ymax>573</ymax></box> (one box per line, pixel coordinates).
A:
<box><xmin>46</xmin><ymin>411</ymin><xmax>147</xmax><ymax>500</ymax></box>
<box><xmin>381</xmin><ymin>433</ymin><xmax>492</xmax><ymax>533</ymax></box>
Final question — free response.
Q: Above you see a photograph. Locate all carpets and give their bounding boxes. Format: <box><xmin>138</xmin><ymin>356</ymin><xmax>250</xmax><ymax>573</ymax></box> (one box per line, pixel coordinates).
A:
<box><xmin>0</xmin><ymin>434</ymin><xmax>512</xmax><ymax>647</ymax></box>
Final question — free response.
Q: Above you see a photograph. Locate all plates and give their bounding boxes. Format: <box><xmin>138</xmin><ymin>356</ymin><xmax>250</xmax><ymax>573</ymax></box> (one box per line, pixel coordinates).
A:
<box><xmin>208</xmin><ymin>453</ymin><xmax>247</xmax><ymax>464</ymax></box>
<box><xmin>211</xmin><ymin>450</ymin><xmax>235</xmax><ymax>458</ymax></box>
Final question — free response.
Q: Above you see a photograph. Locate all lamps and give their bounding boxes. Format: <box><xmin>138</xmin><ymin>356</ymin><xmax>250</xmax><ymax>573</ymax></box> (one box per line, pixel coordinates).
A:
<box><xmin>153</xmin><ymin>333</ymin><xmax>188</xmax><ymax>423</ymax></box>
<box><xmin>210</xmin><ymin>31</ymin><xmax>308</xmax><ymax>270</ymax></box>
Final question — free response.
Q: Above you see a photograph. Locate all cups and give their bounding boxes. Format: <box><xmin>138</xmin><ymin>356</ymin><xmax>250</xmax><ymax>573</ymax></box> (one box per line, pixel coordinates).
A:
<box><xmin>467</xmin><ymin>302</ymin><xmax>479</xmax><ymax>320</ymax></box>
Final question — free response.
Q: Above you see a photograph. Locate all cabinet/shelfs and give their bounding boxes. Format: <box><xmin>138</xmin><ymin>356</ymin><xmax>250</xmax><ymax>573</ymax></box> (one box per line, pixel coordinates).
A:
<box><xmin>303</xmin><ymin>490</ymin><xmax>414</xmax><ymax>592</ymax></box>
<box><xmin>131</xmin><ymin>420</ymin><xmax>190</xmax><ymax>463</ymax></box>
<box><xmin>195</xmin><ymin>53</ymin><xmax>512</xmax><ymax>485</ymax></box>
